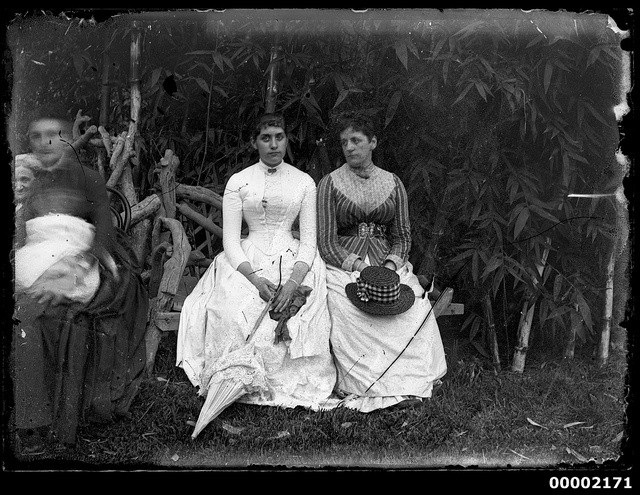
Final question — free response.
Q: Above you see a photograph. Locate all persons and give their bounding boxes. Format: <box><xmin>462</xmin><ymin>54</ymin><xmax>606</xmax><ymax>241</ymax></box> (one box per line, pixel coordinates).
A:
<box><xmin>317</xmin><ymin>110</ymin><xmax>448</xmax><ymax>414</ymax></box>
<box><xmin>11</xmin><ymin>153</ymin><xmax>41</xmax><ymax>251</ymax></box>
<box><xmin>14</xmin><ymin>106</ymin><xmax>115</xmax><ymax>456</ymax></box>
<box><xmin>175</xmin><ymin>113</ymin><xmax>342</xmax><ymax>413</ymax></box>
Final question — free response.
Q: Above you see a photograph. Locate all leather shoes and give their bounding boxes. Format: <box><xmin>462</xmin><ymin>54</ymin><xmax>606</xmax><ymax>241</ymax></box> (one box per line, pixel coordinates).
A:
<box><xmin>19</xmin><ymin>436</ymin><xmax>47</xmax><ymax>455</ymax></box>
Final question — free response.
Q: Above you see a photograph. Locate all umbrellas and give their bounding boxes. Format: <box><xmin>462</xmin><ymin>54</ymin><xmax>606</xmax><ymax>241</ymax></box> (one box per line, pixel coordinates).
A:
<box><xmin>191</xmin><ymin>285</ymin><xmax>281</xmax><ymax>440</ymax></box>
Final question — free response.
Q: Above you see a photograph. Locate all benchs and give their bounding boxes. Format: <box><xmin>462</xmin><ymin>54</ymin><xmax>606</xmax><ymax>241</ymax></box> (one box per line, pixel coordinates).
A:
<box><xmin>140</xmin><ymin>147</ymin><xmax>466</xmax><ymax>369</ymax></box>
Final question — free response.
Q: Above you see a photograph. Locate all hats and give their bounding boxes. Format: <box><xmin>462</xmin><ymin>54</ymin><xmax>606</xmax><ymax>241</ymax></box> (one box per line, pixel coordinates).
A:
<box><xmin>345</xmin><ymin>266</ymin><xmax>415</xmax><ymax>315</ymax></box>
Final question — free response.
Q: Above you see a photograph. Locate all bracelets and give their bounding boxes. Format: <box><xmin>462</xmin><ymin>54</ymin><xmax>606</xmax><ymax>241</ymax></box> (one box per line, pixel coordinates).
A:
<box><xmin>289</xmin><ymin>278</ymin><xmax>300</xmax><ymax>287</ymax></box>
